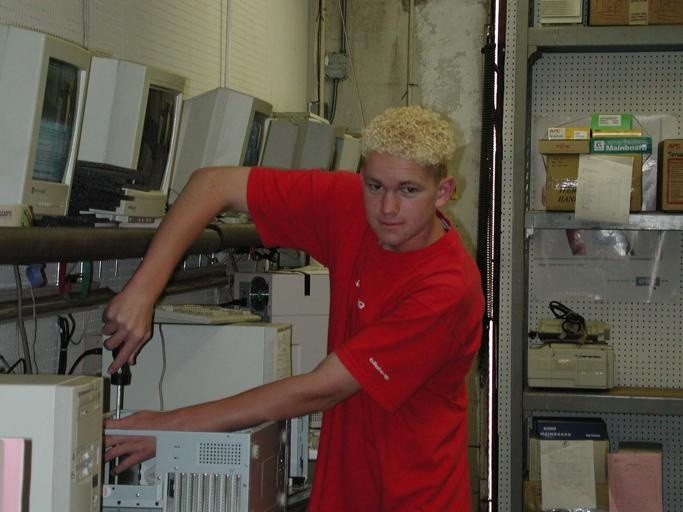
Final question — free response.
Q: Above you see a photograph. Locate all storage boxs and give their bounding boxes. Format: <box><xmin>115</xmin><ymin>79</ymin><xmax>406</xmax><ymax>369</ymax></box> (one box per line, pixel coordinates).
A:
<box><xmin>656</xmin><ymin>139</ymin><xmax>682</xmax><ymax>214</ymax></box>
<box><xmin>541</xmin><ymin>154</ymin><xmax>644</xmax><ymax>213</ymax></box>
<box><xmin>587</xmin><ymin>0</ymin><xmax>682</xmax><ymax>28</ymax></box>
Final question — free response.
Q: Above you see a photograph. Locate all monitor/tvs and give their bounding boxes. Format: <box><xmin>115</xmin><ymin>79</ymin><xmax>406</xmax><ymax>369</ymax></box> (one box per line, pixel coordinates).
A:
<box><xmin>167</xmin><ymin>85</ymin><xmax>274</xmax><ymax>225</ymax></box>
<box><xmin>0</xmin><ymin>24</ymin><xmax>94</xmax><ymax>219</ymax></box>
<box><xmin>261</xmin><ymin>111</ymin><xmax>336</xmax><ymax>170</ymax></box>
<box><xmin>73</xmin><ymin>54</ymin><xmax>187</xmax><ymax>228</ymax></box>
<box><xmin>334</xmin><ymin>133</ymin><xmax>363</xmax><ymax>173</ymax></box>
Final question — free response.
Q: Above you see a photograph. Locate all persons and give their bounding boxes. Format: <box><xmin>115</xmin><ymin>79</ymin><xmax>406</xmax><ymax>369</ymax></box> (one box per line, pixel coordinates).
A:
<box><xmin>96</xmin><ymin>103</ymin><xmax>488</xmax><ymax>512</ymax></box>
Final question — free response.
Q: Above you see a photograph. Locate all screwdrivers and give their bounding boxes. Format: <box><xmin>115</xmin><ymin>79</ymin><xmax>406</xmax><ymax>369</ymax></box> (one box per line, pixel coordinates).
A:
<box><xmin>110</xmin><ymin>340</ymin><xmax>132</xmax><ymax>485</ymax></box>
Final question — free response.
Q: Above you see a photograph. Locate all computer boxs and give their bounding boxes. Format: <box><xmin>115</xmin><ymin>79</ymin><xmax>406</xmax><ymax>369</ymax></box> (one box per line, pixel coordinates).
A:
<box><xmin>103</xmin><ymin>408</ymin><xmax>290</xmax><ymax>512</ymax></box>
<box><xmin>1</xmin><ymin>373</ymin><xmax>104</xmax><ymax>511</ymax></box>
<box><xmin>100</xmin><ymin>323</ymin><xmax>294</xmax><ymax>411</ymax></box>
<box><xmin>232</xmin><ymin>270</ymin><xmax>332</xmax><ymax>429</ymax></box>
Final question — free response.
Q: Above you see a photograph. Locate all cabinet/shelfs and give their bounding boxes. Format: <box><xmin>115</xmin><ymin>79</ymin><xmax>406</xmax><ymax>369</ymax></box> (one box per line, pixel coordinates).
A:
<box><xmin>498</xmin><ymin>0</ymin><xmax>682</xmax><ymax>512</ymax></box>
